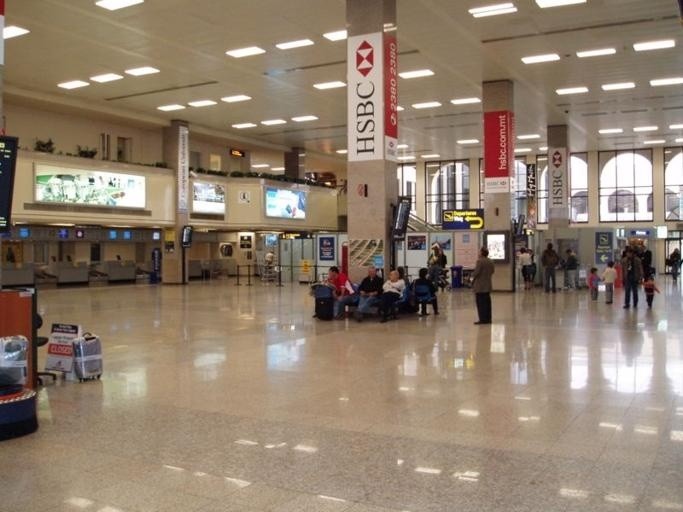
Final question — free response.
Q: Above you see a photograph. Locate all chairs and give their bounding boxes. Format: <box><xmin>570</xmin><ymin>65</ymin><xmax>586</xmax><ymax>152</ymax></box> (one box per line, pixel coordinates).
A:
<box><xmin>312</xmin><ymin>278</ymin><xmax>439</xmax><ymax>323</ymax></box>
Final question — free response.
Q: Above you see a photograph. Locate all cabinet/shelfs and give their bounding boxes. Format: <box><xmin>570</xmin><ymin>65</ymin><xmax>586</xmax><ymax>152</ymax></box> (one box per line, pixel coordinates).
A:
<box><xmin>261</xmin><ymin>264</ymin><xmax>276</xmax><ymax>281</ymax></box>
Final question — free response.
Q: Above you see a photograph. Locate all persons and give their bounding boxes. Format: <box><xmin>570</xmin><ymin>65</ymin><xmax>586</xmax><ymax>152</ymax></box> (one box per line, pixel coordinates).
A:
<box><xmin>379</xmin><ymin>270</ymin><xmax>406</xmax><ymax>323</ymax></box>
<box><xmin>397</xmin><ymin>267</ymin><xmax>409</xmax><ymax>287</ymax></box>
<box><xmin>329</xmin><ymin>266</ymin><xmax>356</xmax><ymax>320</ymax></box>
<box><xmin>410</xmin><ymin>268</ymin><xmax>440</xmax><ymax>315</ymax></box>
<box><xmin>427</xmin><ymin>238</ymin><xmax>683</xmax><ymax>325</ymax></box>
<box><xmin>353</xmin><ymin>267</ymin><xmax>384</xmax><ymax>322</ymax></box>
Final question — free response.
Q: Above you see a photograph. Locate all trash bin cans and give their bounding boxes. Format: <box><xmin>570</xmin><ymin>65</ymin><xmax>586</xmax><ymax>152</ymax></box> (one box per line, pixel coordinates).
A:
<box><xmin>450</xmin><ymin>265</ymin><xmax>463</xmax><ymax>289</ymax></box>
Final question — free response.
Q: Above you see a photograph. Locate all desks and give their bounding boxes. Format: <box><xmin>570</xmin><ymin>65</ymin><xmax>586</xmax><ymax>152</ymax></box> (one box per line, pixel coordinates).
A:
<box><xmin>463</xmin><ymin>268</ymin><xmax>475</xmax><ymax>284</ymax></box>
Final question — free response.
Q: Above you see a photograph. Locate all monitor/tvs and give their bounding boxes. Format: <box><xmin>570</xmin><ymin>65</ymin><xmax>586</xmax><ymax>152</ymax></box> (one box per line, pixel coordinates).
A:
<box><xmin>512</xmin><ymin>215</ymin><xmax>525</xmax><ymax>236</ymax></box>
<box><xmin>18</xmin><ymin>227</ymin><xmax>31</xmax><ymax>239</ymax></box>
<box><xmin>58</xmin><ymin>229</ymin><xmax>69</xmax><ymax>238</ymax></box>
<box><xmin>264</xmin><ymin>234</ymin><xmax>279</xmax><ymax>248</ymax></box>
<box><xmin>74</xmin><ymin>229</ymin><xmax>84</xmax><ymax>239</ymax></box>
<box><xmin>390</xmin><ymin>196</ymin><xmax>411</xmax><ymax>241</ymax></box>
<box><xmin>122</xmin><ymin>230</ymin><xmax>132</xmax><ymax>240</ymax></box>
<box><xmin>152</xmin><ymin>232</ymin><xmax>160</xmax><ymax>240</ymax></box>
<box><xmin>107</xmin><ymin>230</ymin><xmax>118</xmax><ymax>240</ymax></box>
<box><xmin>182</xmin><ymin>226</ymin><xmax>193</xmax><ymax>248</ymax></box>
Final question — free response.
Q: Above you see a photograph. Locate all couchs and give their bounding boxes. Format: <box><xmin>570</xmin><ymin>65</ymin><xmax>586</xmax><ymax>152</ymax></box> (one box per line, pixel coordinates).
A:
<box><xmin>45</xmin><ymin>262</ymin><xmax>88</xmax><ymax>288</ymax></box>
<box><xmin>95</xmin><ymin>258</ymin><xmax>136</xmax><ymax>285</ymax></box>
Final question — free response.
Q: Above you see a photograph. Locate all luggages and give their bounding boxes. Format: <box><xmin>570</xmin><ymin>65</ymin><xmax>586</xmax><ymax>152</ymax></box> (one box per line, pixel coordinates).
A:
<box><xmin>314</xmin><ymin>285</ymin><xmax>334</xmax><ymax>320</ymax></box>
<box><xmin>72</xmin><ymin>331</ymin><xmax>102</xmax><ymax>383</ymax></box>
